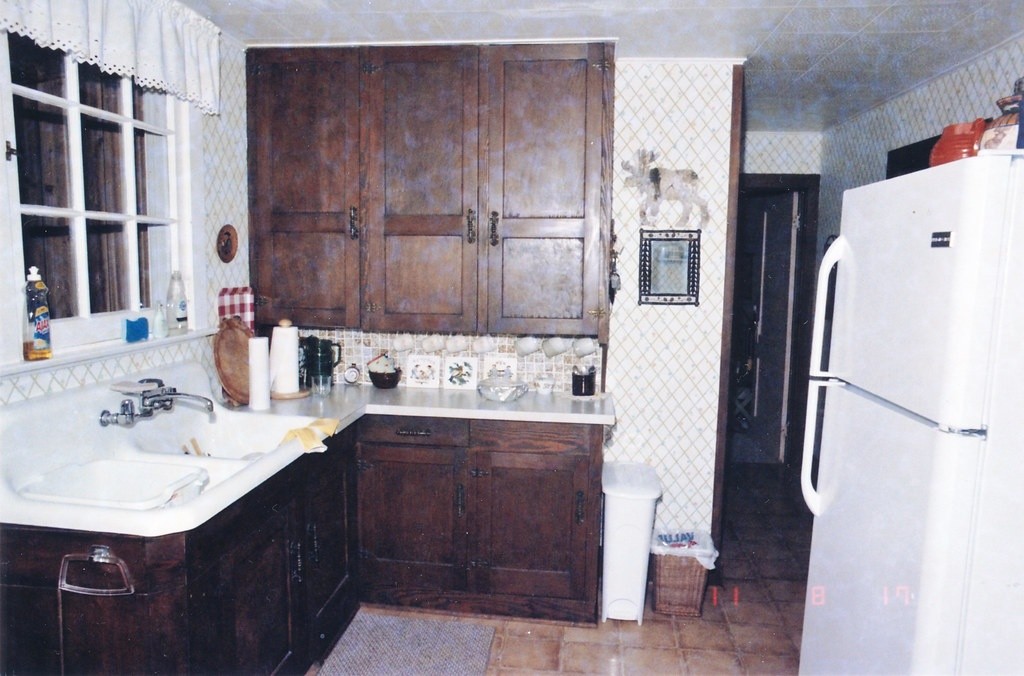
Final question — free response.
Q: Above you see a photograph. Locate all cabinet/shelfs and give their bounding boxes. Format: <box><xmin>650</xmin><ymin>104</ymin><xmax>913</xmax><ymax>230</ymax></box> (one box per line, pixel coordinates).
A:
<box><xmin>245</xmin><ymin>41</ymin><xmax>610</xmax><ymax>337</ymax></box>
<box><xmin>0</xmin><ymin>414</ymin><xmax>603</xmax><ymax>675</ymax></box>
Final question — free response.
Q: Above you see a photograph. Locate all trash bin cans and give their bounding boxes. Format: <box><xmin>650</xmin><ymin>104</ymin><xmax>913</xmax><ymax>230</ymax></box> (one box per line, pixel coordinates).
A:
<box><xmin>651</xmin><ymin>529</ymin><xmax>716</xmax><ymax>617</ymax></box>
<box><xmin>601</xmin><ymin>461</ymin><xmax>662</xmax><ymax>626</ymax></box>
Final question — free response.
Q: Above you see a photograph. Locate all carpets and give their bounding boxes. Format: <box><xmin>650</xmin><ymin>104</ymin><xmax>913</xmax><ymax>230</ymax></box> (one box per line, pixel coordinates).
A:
<box><xmin>317</xmin><ymin>613</ymin><xmax>496</xmax><ymax>675</ymax></box>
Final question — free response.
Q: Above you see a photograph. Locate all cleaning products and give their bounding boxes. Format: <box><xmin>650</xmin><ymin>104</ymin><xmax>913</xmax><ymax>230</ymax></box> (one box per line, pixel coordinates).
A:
<box><xmin>21</xmin><ymin>266</ymin><xmax>51</xmax><ymax>361</ymax></box>
<box><xmin>122</xmin><ymin>301</ymin><xmax>148</xmax><ymax>343</ymax></box>
<box><xmin>151</xmin><ymin>270</ymin><xmax>189</xmax><ymax>340</ymax></box>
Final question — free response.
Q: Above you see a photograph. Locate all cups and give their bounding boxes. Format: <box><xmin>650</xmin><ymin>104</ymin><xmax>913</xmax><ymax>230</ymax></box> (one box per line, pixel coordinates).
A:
<box><xmin>572</xmin><ymin>372</ymin><xmax>596</xmax><ymax>396</ymax></box>
<box><xmin>446</xmin><ymin>331</ymin><xmax>467</xmax><ymax>353</ymax></box>
<box><xmin>473</xmin><ymin>333</ymin><xmax>495</xmax><ymax>353</ymax></box>
<box><xmin>573</xmin><ymin>335</ymin><xmax>596</xmax><ymax>357</ymax></box>
<box><xmin>312</xmin><ymin>376</ymin><xmax>331</xmax><ymax>395</ymax></box>
<box><xmin>423</xmin><ymin>330</ymin><xmax>444</xmax><ymax>352</ymax></box>
<box><xmin>515</xmin><ymin>334</ymin><xmax>539</xmax><ymax>357</ymax></box>
<box><xmin>394</xmin><ymin>330</ymin><xmax>413</xmax><ymax>352</ymax></box>
<box><xmin>542</xmin><ymin>334</ymin><xmax>566</xmax><ymax>356</ymax></box>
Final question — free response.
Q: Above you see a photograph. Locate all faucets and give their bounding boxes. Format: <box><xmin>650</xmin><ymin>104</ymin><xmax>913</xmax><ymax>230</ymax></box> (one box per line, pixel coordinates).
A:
<box><xmin>143</xmin><ymin>389</ymin><xmax>214</xmax><ymax>413</ymax></box>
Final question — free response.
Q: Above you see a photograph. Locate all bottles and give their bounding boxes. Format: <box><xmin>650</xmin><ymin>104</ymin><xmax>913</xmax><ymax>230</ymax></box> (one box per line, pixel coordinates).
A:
<box><xmin>269</xmin><ymin>320</ymin><xmax>299</xmax><ymax>394</ymax></box>
<box><xmin>169</xmin><ymin>272</ymin><xmax>188</xmax><ymax>336</ymax></box>
<box><xmin>153</xmin><ymin>302</ymin><xmax>169</xmax><ymax>339</ymax></box>
<box><xmin>22</xmin><ymin>267</ymin><xmax>52</xmax><ymax>360</ymax></box>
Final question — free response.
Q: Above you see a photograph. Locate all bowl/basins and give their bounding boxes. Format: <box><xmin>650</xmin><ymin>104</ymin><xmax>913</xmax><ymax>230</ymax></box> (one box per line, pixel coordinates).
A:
<box><xmin>369</xmin><ymin>368</ymin><xmax>402</xmax><ymax>389</ymax></box>
<box><xmin>479</xmin><ymin>378</ymin><xmax>527</xmax><ymax>401</ymax></box>
<box><xmin>534</xmin><ymin>378</ymin><xmax>555</xmax><ymax>394</ymax></box>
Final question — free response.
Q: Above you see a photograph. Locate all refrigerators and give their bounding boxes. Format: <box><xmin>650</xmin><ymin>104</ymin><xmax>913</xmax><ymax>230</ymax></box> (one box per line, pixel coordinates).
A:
<box><xmin>801</xmin><ymin>149</ymin><xmax>1024</xmax><ymax>676</ymax></box>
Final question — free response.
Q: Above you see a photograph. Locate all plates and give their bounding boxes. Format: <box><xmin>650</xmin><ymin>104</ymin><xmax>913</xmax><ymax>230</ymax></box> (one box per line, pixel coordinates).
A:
<box><xmin>214</xmin><ymin>318</ymin><xmax>253</xmax><ymax>404</ymax></box>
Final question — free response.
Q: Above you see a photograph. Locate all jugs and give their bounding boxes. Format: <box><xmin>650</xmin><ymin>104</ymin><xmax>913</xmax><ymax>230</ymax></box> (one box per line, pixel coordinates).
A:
<box><xmin>306</xmin><ymin>340</ymin><xmax>341</xmax><ymax>386</ymax></box>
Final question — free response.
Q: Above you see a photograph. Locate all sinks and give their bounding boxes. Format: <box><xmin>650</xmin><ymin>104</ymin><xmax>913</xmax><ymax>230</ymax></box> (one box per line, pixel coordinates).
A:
<box><xmin>22</xmin><ymin>459</ymin><xmax>246</xmax><ymax>504</ymax></box>
<box><xmin>131</xmin><ymin>412</ymin><xmax>314</xmax><ymax>457</ymax></box>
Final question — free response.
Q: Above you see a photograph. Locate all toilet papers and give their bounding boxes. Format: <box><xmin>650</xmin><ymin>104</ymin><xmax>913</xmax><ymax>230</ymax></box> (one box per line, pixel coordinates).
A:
<box><xmin>269</xmin><ymin>326</ymin><xmax>300</xmax><ymax>393</ymax></box>
<box><xmin>248</xmin><ymin>337</ymin><xmax>271</xmax><ymax>412</ymax></box>
